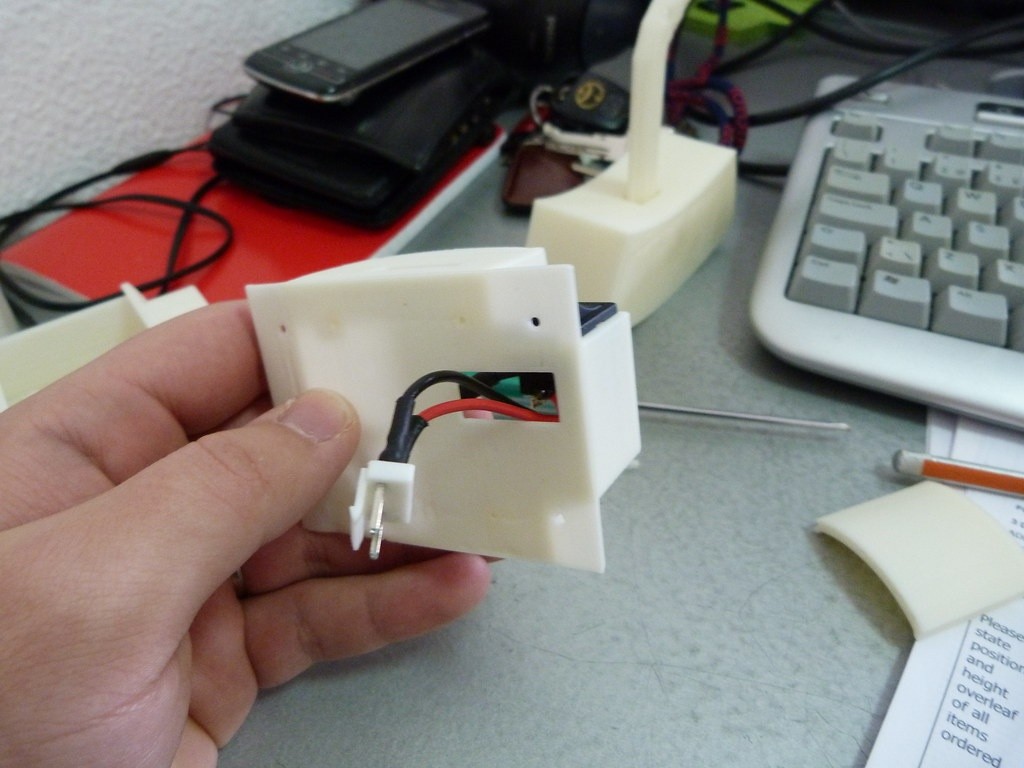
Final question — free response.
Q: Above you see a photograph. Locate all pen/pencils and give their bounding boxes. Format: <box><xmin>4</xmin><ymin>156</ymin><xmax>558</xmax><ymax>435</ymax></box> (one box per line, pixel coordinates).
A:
<box><xmin>891</xmin><ymin>449</ymin><xmax>1024</xmax><ymax>501</ymax></box>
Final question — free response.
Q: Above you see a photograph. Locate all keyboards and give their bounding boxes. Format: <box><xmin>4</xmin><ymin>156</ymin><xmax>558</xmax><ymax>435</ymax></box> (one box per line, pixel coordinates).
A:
<box><xmin>748</xmin><ymin>74</ymin><xmax>1023</xmax><ymax>435</ymax></box>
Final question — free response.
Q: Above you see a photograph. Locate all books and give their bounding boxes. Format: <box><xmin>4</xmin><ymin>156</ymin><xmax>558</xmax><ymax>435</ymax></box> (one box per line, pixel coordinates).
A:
<box><xmin>0</xmin><ymin>125</ymin><xmax>507</xmax><ymax>305</ymax></box>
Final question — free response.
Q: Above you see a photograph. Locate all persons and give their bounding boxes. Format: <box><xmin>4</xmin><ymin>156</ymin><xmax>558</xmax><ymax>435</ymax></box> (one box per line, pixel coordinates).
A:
<box><xmin>0</xmin><ymin>300</ymin><xmax>502</xmax><ymax>768</ymax></box>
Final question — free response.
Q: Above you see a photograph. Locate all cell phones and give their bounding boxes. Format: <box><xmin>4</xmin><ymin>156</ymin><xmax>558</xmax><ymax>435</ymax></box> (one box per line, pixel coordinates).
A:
<box><xmin>242</xmin><ymin>0</ymin><xmax>491</xmax><ymax>103</ymax></box>
